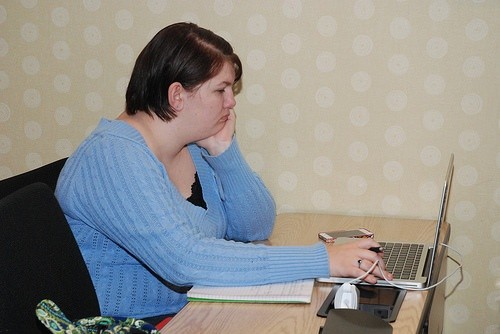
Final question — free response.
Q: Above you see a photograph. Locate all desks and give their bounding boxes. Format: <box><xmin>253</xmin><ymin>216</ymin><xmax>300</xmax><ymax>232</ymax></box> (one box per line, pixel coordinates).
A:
<box><xmin>155</xmin><ymin>212</ymin><xmax>451</xmax><ymax>334</ymax></box>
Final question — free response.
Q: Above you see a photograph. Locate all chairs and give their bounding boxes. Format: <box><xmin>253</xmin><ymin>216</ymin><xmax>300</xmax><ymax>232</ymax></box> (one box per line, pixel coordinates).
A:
<box><xmin>0</xmin><ymin>158</ymin><xmax>103</xmax><ymax>334</ymax></box>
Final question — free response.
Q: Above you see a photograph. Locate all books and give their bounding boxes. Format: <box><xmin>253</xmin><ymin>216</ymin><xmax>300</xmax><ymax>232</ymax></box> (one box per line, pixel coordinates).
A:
<box><xmin>187</xmin><ymin>278</ymin><xmax>315</xmax><ymax>303</ymax></box>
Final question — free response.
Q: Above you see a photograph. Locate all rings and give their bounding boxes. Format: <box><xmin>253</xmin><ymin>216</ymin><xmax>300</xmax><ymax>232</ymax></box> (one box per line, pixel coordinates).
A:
<box><xmin>358</xmin><ymin>258</ymin><xmax>364</xmax><ymax>269</ymax></box>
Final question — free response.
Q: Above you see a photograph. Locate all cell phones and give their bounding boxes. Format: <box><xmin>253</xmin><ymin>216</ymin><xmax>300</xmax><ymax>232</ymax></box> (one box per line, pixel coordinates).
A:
<box><xmin>317</xmin><ymin>227</ymin><xmax>374</xmax><ymax>243</ymax></box>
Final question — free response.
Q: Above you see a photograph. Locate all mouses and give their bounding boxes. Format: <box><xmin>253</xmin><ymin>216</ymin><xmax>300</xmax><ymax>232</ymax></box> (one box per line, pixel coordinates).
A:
<box><xmin>334</xmin><ymin>283</ymin><xmax>360</xmax><ymax>311</ymax></box>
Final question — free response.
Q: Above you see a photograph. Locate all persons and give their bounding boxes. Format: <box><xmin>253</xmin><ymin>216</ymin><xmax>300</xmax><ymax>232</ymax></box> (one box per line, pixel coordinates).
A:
<box><xmin>54</xmin><ymin>22</ymin><xmax>393</xmax><ymax>334</ymax></box>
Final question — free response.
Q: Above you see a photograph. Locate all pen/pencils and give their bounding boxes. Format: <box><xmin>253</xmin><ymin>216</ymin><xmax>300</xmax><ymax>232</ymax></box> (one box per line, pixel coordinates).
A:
<box><xmin>368</xmin><ymin>247</ymin><xmax>385</xmax><ymax>253</ymax></box>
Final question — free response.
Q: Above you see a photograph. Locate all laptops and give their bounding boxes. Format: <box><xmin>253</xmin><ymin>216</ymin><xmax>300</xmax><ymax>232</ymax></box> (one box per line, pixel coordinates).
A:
<box><xmin>316</xmin><ymin>153</ymin><xmax>454</xmax><ymax>290</ymax></box>
